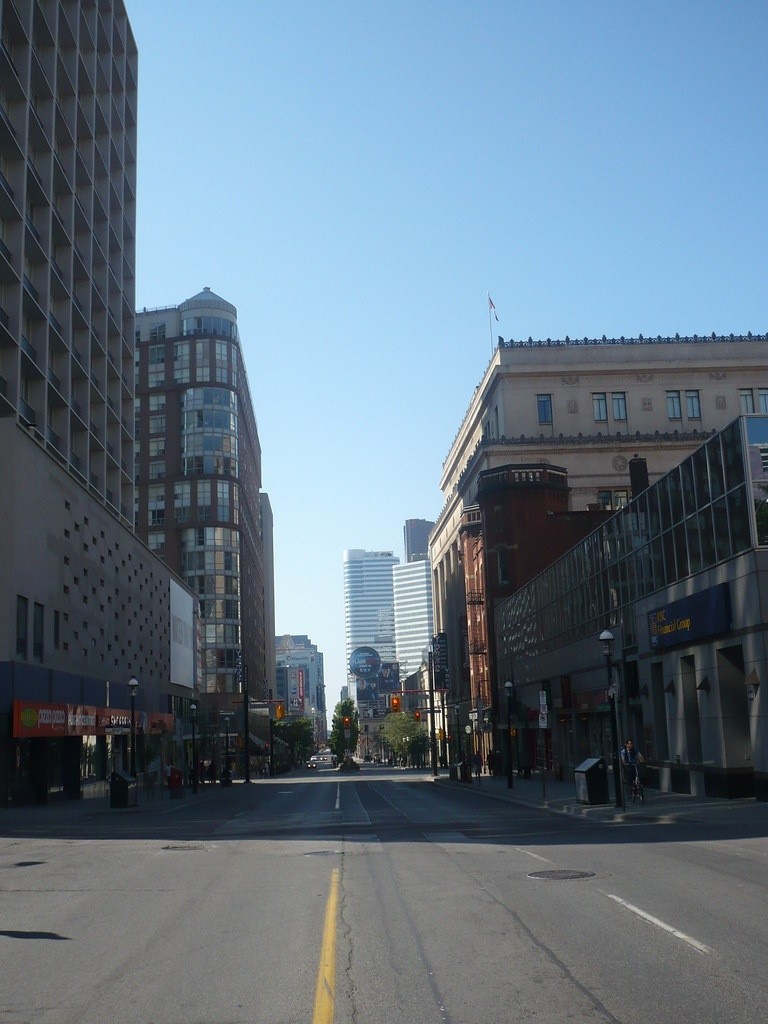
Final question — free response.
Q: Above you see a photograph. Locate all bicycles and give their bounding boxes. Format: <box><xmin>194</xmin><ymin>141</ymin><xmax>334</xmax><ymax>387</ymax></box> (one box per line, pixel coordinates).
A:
<box><xmin>625</xmin><ymin>761</ymin><xmax>645</xmax><ymax>804</ymax></box>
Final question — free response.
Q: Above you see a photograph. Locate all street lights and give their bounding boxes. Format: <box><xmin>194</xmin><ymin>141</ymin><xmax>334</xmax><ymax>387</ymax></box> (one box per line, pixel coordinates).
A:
<box><xmin>189</xmin><ymin>704</ymin><xmax>200</xmax><ymax>793</ymax></box>
<box><xmin>599</xmin><ymin>626</ymin><xmax>626</xmax><ymax>808</ymax></box>
<box><xmin>127</xmin><ymin>677</ymin><xmax>138</xmax><ymax>781</ymax></box>
<box><xmin>504</xmin><ymin>678</ymin><xmax>517</xmax><ymax>789</ymax></box>
<box><xmin>223</xmin><ymin>717</ymin><xmax>231</xmax><ymax>769</ymax></box>
<box><xmin>454</xmin><ymin>704</ymin><xmax>463</xmax><ymax>766</ymax></box>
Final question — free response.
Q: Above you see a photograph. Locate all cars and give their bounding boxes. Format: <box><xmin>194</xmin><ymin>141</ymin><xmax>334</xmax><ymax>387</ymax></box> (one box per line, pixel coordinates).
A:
<box><xmin>363</xmin><ymin>756</ymin><xmax>371</xmax><ymax>763</ymax></box>
<box><xmin>308</xmin><ymin>762</ymin><xmax>316</xmax><ymax>768</ymax></box>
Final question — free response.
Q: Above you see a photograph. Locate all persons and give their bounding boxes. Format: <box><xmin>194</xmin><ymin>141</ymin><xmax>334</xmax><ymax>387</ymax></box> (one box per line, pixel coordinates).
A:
<box><xmin>379</xmin><ymin>666</ymin><xmax>395</xmax><ymax>684</ymax></box>
<box><xmin>357</xmin><ymin>678</ymin><xmax>376</xmax><ymax>701</ymax></box>
<box><xmin>620</xmin><ymin>739</ymin><xmax>645</xmax><ymax>792</ymax></box>
<box><xmin>474</xmin><ymin>752</ymin><xmax>483</xmax><ymax>779</ymax></box>
<box><xmin>487</xmin><ymin>750</ymin><xmax>494</xmax><ymax>775</ymax></box>
<box><xmin>196</xmin><ymin>760</ymin><xmax>238</xmax><ymax>784</ymax></box>
<box><xmin>333</xmin><ymin>757</ymin><xmax>337</xmax><ymax>768</ymax></box>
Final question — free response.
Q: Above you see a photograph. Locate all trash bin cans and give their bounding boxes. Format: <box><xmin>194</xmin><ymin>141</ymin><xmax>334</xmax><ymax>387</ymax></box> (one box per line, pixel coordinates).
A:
<box><xmin>572</xmin><ymin>757</ymin><xmax>612</xmax><ymax>806</ymax></box>
<box><xmin>110</xmin><ymin>769</ymin><xmax>138</xmax><ymax>809</ymax></box>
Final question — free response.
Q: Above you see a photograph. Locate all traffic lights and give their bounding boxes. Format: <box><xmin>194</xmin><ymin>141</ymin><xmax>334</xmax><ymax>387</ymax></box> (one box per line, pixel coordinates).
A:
<box><xmin>344</xmin><ymin>717</ymin><xmax>350</xmax><ymax>726</ymax></box>
<box><xmin>415</xmin><ymin>711</ymin><xmax>420</xmax><ymax>722</ymax></box>
<box><xmin>391</xmin><ymin>696</ymin><xmax>400</xmax><ymax>713</ymax></box>
